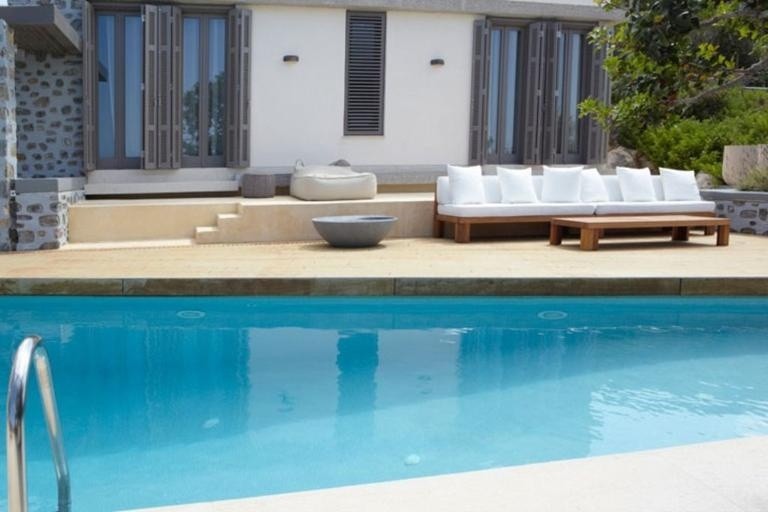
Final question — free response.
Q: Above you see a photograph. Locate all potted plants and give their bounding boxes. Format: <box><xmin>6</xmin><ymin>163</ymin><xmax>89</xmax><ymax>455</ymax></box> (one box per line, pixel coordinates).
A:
<box><xmin>718</xmin><ymin>114</ymin><xmax>767</xmax><ymax>191</ymax></box>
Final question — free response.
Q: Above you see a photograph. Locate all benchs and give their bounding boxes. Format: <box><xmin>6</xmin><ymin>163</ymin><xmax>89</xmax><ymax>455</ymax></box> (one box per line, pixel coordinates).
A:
<box><xmin>241</xmin><ymin>164</ymin><xmax>447</xmax><ymax>199</ymax></box>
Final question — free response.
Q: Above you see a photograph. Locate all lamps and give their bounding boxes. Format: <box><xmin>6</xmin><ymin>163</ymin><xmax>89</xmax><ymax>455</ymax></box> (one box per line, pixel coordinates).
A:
<box><xmin>282</xmin><ymin>55</ymin><xmax>300</xmax><ymax>67</ymax></box>
<box><xmin>430</xmin><ymin>58</ymin><xmax>444</xmax><ymax>71</ymax></box>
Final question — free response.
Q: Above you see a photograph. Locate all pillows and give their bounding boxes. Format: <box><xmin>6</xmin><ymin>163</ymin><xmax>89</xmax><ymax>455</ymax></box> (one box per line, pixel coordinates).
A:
<box><xmin>447</xmin><ymin>159</ymin><xmax>701</xmax><ymax>204</ymax></box>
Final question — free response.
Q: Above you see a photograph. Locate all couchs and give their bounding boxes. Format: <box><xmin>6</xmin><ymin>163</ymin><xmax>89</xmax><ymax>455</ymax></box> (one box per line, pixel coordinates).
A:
<box><xmin>433</xmin><ymin>175</ymin><xmax>717</xmax><ymax>244</ymax></box>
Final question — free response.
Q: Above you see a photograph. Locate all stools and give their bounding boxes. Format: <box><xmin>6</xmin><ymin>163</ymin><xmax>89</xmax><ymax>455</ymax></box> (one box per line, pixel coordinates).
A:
<box><xmin>290</xmin><ymin>155</ymin><xmax>378</xmax><ymax>200</ymax></box>
